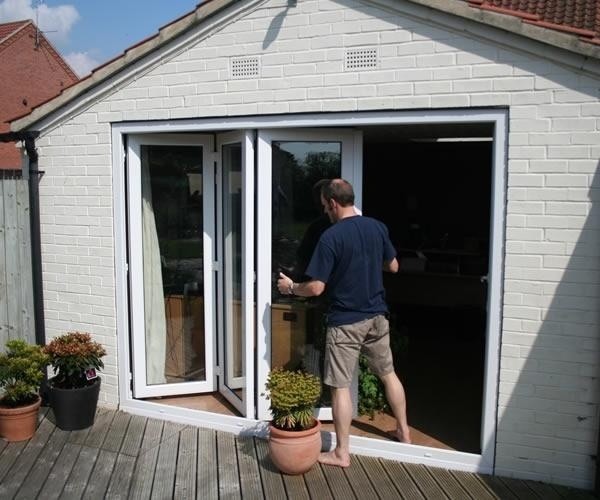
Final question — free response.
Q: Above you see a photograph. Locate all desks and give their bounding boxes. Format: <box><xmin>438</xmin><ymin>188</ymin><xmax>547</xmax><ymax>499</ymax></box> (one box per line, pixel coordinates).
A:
<box><xmin>163</xmin><ymin>293</ymin><xmax>322</xmax><ymax>387</ymax></box>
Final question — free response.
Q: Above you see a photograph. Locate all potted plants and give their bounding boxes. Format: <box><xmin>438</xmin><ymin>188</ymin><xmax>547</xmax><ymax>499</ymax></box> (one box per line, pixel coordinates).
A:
<box><xmin>265</xmin><ymin>367</ymin><xmax>323</xmax><ymax>478</ymax></box>
<box><xmin>1</xmin><ymin>331</ymin><xmax>107</xmax><ymax>445</ymax></box>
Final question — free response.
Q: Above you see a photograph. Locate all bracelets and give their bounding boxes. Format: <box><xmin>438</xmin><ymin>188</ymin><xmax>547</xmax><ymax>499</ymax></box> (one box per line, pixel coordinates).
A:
<box><xmin>286</xmin><ymin>282</ymin><xmax>295</xmax><ymax>297</ymax></box>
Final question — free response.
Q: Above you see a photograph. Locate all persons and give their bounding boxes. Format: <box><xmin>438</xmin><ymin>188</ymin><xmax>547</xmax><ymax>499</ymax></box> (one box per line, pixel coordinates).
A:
<box><xmin>275</xmin><ymin>178</ymin><xmax>333</xmax><ymax>407</ymax></box>
<box><xmin>276</xmin><ymin>177</ymin><xmax>412</xmax><ymax>469</ymax></box>
